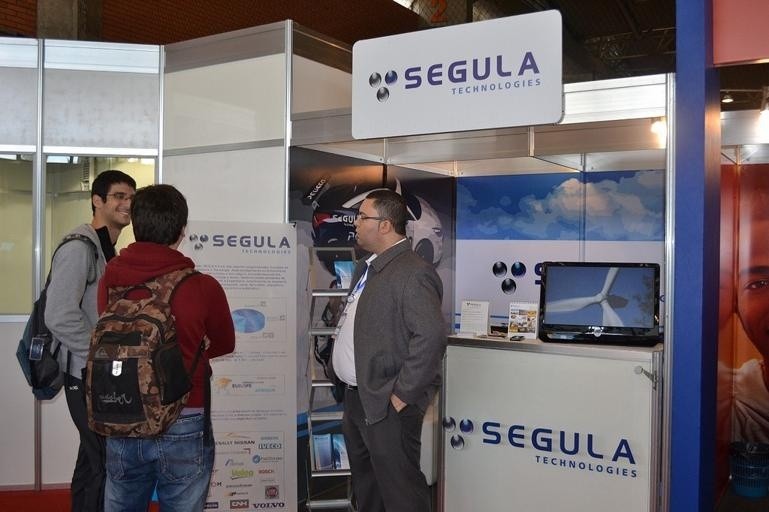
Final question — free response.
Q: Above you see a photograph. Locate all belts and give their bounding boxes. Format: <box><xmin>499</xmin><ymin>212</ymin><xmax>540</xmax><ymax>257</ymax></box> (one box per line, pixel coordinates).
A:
<box><xmin>345</xmin><ymin>383</ymin><xmax>359</xmax><ymax>393</ymax></box>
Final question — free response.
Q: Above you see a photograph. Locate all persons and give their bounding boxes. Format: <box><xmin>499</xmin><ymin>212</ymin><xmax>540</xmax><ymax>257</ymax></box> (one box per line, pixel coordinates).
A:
<box><xmin>96</xmin><ymin>184</ymin><xmax>234</xmax><ymax>512</ymax></box>
<box><xmin>728</xmin><ymin>164</ymin><xmax>769</xmax><ymax>447</ymax></box>
<box><xmin>326</xmin><ymin>189</ymin><xmax>446</xmax><ymax>511</ymax></box>
<box><xmin>44</xmin><ymin>169</ymin><xmax>137</xmax><ymax>511</ymax></box>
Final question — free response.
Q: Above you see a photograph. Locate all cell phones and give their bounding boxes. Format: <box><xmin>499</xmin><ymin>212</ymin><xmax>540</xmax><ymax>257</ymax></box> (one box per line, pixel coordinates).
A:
<box><xmin>510</xmin><ymin>334</ymin><xmax>525</xmax><ymax>342</ymax></box>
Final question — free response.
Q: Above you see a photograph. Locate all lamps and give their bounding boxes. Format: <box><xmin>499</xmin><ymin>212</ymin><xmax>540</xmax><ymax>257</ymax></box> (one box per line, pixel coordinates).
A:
<box><xmin>721</xmin><ymin>89</ymin><xmax>735</xmax><ymax>104</ymax></box>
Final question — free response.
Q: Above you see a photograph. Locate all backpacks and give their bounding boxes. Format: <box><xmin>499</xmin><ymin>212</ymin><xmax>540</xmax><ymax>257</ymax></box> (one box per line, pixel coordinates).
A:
<box><xmin>13</xmin><ymin>233</ymin><xmax>99</xmax><ymax>402</ymax></box>
<box><xmin>84</xmin><ymin>267</ymin><xmax>205</xmax><ymax>440</ymax></box>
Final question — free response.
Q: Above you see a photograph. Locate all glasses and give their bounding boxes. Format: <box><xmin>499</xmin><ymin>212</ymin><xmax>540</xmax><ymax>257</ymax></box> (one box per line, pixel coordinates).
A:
<box><xmin>101</xmin><ymin>191</ymin><xmax>135</xmax><ymax>202</ymax></box>
<box><xmin>357</xmin><ymin>214</ymin><xmax>388</xmax><ymax>222</ymax></box>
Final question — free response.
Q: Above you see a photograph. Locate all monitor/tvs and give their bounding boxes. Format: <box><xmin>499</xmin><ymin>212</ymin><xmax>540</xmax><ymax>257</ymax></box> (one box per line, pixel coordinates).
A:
<box><xmin>536</xmin><ymin>260</ymin><xmax>661</xmax><ymax>345</ymax></box>
